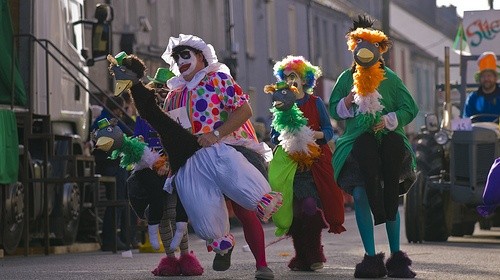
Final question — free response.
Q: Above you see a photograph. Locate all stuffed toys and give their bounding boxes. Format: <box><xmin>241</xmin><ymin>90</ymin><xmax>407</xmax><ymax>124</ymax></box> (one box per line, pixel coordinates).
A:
<box><xmin>346</xmin><ymin>26</ymin><xmax>406</xmax><ymax>225</ymax></box>
<box><xmin>264</xmin><ymin>81</ymin><xmax>347</xmax><ymax>235</ymax></box>
<box><xmin>96</xmin><ymin>119</ymin><xmax>188</xmax><ymax>251</ymax></box>
<box><xmin>106</xmin><ymin>51</ymin><xmax>283</xmax><ymax>257</ymax></box>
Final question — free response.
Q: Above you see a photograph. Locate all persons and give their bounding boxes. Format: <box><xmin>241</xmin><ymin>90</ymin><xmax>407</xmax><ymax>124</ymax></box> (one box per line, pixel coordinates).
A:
<box><xmin>90</xmin><ymin>13</ymin><xmax>500</xmax><ymax>280</ymax></box>
<box><xmin>162</xmin><ymin>34</ymin><xmax>274</xmax><ymax>279</ymax></box>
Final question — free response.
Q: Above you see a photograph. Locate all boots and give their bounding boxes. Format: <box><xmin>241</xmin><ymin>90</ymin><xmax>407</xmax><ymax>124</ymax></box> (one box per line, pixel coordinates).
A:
<box><xmin>285</xmin><ymin>213</ymin><xmax>308</xmax><ymax>271</ymax></box>
<box><xmin>303</xmin><ymin>209</ymin><xmax>329</xmax><ymax>270</ymax></box>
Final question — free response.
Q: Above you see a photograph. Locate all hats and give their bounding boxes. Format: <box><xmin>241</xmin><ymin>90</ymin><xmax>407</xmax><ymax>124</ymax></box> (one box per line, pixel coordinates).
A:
<box><xmin>161</xmin><ymin>34</ymin><xmax>219</xmax><ymax>77</ymax></box>
<box><xmin>475</xmin><ymin>52</ymin><xmax>500</xmax><ymax>83</ymax></box>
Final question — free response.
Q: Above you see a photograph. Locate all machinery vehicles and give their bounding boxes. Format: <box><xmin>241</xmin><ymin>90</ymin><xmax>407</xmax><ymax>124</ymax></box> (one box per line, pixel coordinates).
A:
<box><xmin>404</xmin><ymin>51</ymin><xmax>499</xmax><ymax>243</ymax></box>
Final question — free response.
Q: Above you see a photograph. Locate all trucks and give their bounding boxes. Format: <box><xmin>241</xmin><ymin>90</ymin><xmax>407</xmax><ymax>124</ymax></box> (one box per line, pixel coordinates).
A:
<box><xmin>0</xmin><ymin>0</ymin><xmax>114</xmax><ymax>257</ymax></box>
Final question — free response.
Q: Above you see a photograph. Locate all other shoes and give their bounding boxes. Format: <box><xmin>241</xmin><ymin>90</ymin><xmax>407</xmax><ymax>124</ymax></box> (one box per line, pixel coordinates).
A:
<box><xmin>354</xmin><ymin>253</ymin><xmax>388</xmax><ymax>279</ymax></box>
<box><xmin>179</xmin><ymin>250</ymin><xmax>204</xmax><ymax>276</ymax></box>
<box><xmin>386</xmin><ymin>249</ymin><xmax>417</xmax><ymax>279</ymax></box>
<box><xmin>213</xmin><ymin>248</ymin><xmax>233</xmax><ymax>272</ymax></box>
<box><xmin>255</xmin><ymin>267</ymin><xmax>275</xmax><ymax>279</ymax></box>
<box><xmin>152</xmin><ymin>256</ymin><xmax>180</xmax><ymax>276</ymax></box>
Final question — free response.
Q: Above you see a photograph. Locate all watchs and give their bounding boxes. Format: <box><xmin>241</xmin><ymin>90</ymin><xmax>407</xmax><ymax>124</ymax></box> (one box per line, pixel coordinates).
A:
<box><xmin>212</xmin><ymin>129</ymin><xmax>221</xmax><ymax>139</ymax></box>
